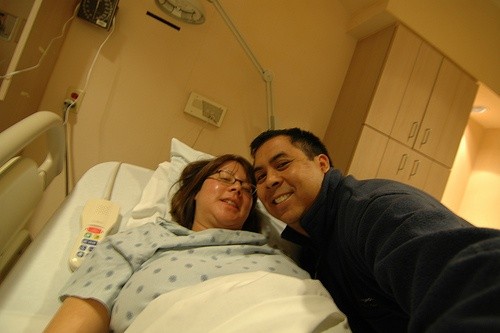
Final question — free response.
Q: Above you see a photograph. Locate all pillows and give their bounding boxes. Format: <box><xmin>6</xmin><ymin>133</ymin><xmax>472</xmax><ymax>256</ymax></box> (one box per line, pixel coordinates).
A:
<box><xmin>126</xmin><ymin>137</ymin><xmax>301</xmax><ymax>263</ymax></box>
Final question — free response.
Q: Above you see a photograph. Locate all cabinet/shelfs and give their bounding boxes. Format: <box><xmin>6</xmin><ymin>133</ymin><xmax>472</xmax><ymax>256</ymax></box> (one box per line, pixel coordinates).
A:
<box><xmin>323</xmin><ymin>22</ymin><xmax>479</xmax><ymax>203</ymax></box>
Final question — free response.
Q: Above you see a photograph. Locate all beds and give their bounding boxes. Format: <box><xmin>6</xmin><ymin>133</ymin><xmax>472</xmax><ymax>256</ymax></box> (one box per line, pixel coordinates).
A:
<box><xmin>0</xmin><ymin>111</ymin><xmax>156</xmax><ymax>333</ymax></box>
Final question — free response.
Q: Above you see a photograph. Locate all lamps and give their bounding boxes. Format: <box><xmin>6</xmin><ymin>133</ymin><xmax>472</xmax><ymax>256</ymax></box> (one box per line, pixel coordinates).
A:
<box><xmin>153</xmin><ymin>0</ymin><xmax>275</xmax><ymax>131</ymax></box>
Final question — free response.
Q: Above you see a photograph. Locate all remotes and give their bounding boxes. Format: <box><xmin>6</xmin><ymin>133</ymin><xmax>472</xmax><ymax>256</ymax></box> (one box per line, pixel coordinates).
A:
<box><xmin>69</xmin><ymin>198</ymin><xmax>121</xmax><ymax>272</ymax></box>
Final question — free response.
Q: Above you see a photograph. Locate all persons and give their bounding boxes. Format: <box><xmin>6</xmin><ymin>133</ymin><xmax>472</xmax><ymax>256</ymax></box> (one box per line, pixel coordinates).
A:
<box><xmin>43</xmin><ymin>153</ymin><xmax>312</xmax><ymax>333</ymax></box>
<box><xmin>249</xmin><ymin>127</ymin><xmax>500</xmax><ymax>333</ymax></box>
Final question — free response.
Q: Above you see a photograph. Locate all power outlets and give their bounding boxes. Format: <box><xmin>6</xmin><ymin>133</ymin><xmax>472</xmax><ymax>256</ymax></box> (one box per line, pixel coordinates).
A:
<box><xmin>64</xmin><ymin>85</ymin><xmax>85</xmax><ymax>114</ymax></box>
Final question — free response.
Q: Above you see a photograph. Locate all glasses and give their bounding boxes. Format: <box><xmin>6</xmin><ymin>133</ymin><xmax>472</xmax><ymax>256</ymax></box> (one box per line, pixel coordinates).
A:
<box><xmin>208</xmin><ymin>169</ymin><xmax>257</xmax><ymax>196</ymax></box>
